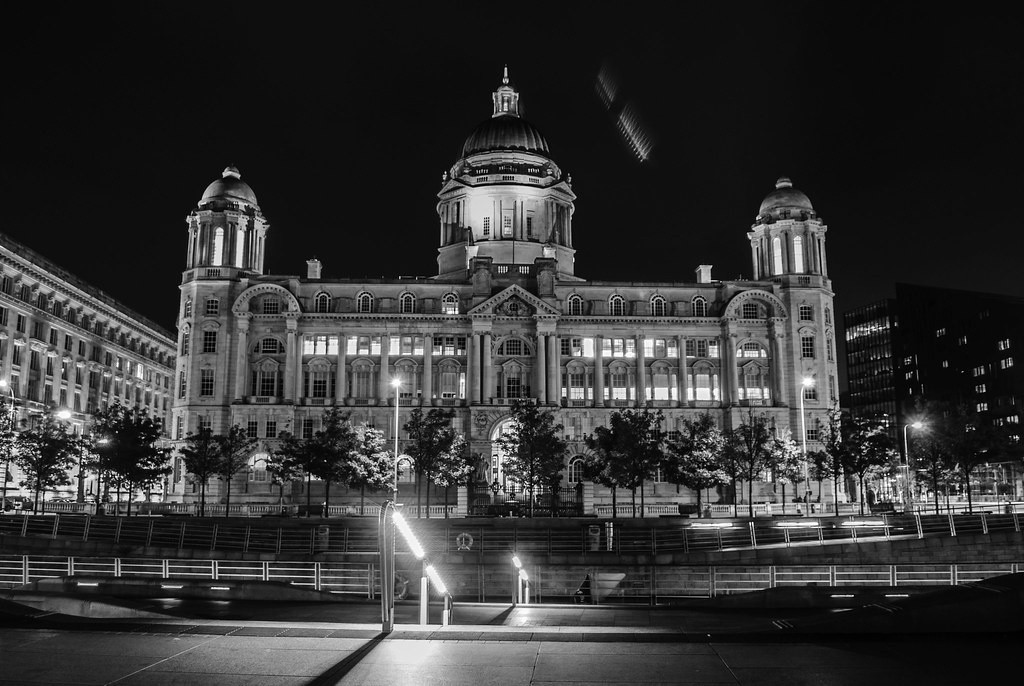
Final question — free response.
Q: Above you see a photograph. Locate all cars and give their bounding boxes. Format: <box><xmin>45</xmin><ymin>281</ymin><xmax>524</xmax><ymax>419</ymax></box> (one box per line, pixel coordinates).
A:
<box><xmin>0</xmin><ymin>498</ymin><xmax>14</xmax><ymax>512</ymax></box>
<box><xmin>300</xmin><ymin>503</ymin><xmax>325</xmax><ymax>518</ymax></box>
<box><xmin>49</xmin><ymin>496</ymin><xmax>76</xmax><ymax>503</ymax></box>
<box><xmin>13</xmin><ymin>496</ymin><xmax>34</xmax><ymax>511</ymax></box>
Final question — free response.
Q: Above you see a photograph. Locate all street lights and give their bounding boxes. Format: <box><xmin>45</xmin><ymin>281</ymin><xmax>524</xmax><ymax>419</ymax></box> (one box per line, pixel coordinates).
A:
<box><xmin>904</xmin><ymin>422</ymin><xmax>922</xmax><ymax>508</ymax></box>
<box><xmin>391</xmin><ymin>378</ymin><xmax>402</xmax><ymax>504</ymax></box>
<box><xmin>0</xmin><ymin>379</ymin><xmax>15</xmax><ymax>510</ymax></box>
<box><xmin>800</xmin><ymin>377</ymin><xmax>814</xmax><ymax>518</ymax></box>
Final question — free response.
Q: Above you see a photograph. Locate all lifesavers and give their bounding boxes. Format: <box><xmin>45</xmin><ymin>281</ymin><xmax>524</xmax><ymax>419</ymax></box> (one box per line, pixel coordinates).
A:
<box><xmin>456</xmin><ymin>532</ymin><xmax>474</xmax><ymax>550</ymax></box>
<box><xmin>394</xmin><ymin>573</ymin><xmax>409</xmax><ymax>600</ymax></box>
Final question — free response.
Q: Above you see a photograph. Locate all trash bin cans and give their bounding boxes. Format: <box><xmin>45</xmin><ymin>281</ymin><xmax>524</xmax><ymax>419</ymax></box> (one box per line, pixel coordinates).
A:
<box><xmin>318</xmin><ymin>525</ymin><xmax>330</xmax><ymax>549</ymax></box>
<box><xmin>346</xmin><ymin>503</ymin><xmax>356</xmax><ymax>517</ymax></box>
<box><xmin>589</xmin><ymin>525</ymin><xmax>601</xmax><ymax>551</ymax></box>
<box><xmin>504</xmin><ymin>501</ymin><xmax>520</xmax><ymax>514</ymax></box>
<box><xmin>99</xmin><ymin>502</ymin><xmax>107</xmax><ymax>515</ymax></box>
